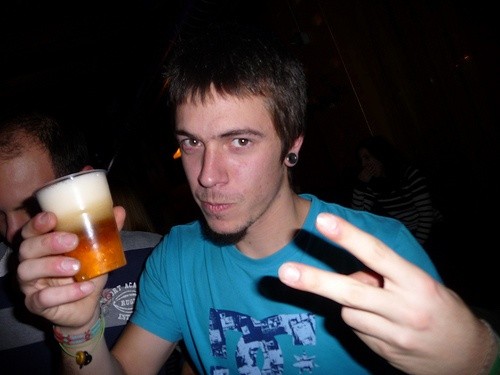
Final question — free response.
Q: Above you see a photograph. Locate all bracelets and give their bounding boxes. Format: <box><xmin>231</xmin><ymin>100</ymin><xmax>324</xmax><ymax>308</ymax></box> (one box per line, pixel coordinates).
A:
<box><xmin>52</xmin><ymin>307</ymin><xmax>106</xmax><ymax>369</ymax></box>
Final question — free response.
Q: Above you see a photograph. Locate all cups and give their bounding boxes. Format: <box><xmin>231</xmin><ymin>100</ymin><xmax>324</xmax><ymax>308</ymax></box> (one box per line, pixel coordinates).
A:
<box><xmin>33</xmin><ymin>168</ymin><xmax>127</xmax><ymax>283</ymax></box>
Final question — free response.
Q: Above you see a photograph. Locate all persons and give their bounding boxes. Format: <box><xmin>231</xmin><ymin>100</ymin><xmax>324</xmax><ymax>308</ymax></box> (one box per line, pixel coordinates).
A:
<box><xmin>350</xmin><ymin>137</ymin><xmax>447</xmax><ymax>258</ymax></box>
<box><xmin>0</xmin><ymin>113</ymin><xmax>199</xmax><ymax>375</ymax></box>
<box><xmin>18</xmin><ymin>39</ymin><xmax>500</xmax><ymax>375</ymax></box>
